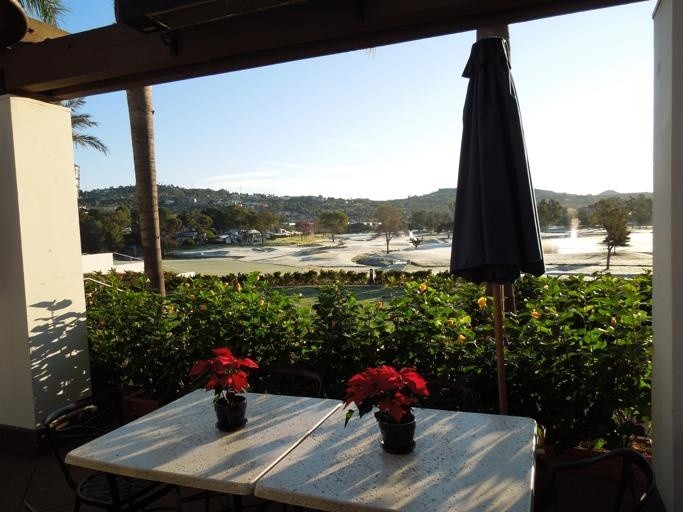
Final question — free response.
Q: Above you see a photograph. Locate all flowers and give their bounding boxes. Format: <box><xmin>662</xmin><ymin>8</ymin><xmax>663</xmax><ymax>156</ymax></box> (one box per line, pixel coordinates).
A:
<box><xmin>341</xmin><ymin>364</ymin><xmax>430</xmax><ymax>427</ymax></box>
<box><xmin>189</xmin><ymin>348</ymin><xmax>259</xmax><ymax>406</ymax></box>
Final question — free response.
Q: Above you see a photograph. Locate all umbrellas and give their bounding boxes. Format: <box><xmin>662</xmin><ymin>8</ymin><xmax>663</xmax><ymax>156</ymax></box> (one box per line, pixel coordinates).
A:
<box><xmin>450</xmin><ymin>37</ymin><xmax>545</xmax><ymax>413</ymax></box>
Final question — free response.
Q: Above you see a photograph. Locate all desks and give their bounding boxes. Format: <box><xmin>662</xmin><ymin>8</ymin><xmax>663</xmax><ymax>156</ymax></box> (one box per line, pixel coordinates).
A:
<box><xmin>63</xmin><ymin>388</ymin><xmax>340</xmax><ymax>496</ymax></box>
<box><xmin>252</xmin><ymin>397</ymin><xmax>538</xmax><ymax>512</ymax></box>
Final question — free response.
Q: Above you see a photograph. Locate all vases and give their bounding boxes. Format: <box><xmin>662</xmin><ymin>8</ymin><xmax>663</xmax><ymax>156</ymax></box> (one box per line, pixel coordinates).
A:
<box><xmin>214</xmin><ymin>395</ymin><xmax>247</xmax><ymax>432</ymax></box>
<box><xmin>376</xmin><ymin>415</ymin><xmax>416</xmax><ymax>455</ymax></box>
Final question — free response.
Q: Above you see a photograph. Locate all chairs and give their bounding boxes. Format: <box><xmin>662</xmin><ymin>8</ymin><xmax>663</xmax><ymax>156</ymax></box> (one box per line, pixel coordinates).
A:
<box><xmin>16</xmin><ymin>395</ymin><xmax>176</xmax><ymax>512</ymax></box>
<box><xmin>537</xmin><ymin>449</ymin><xmax>653</xmax><ymax>510</ymax></box>
